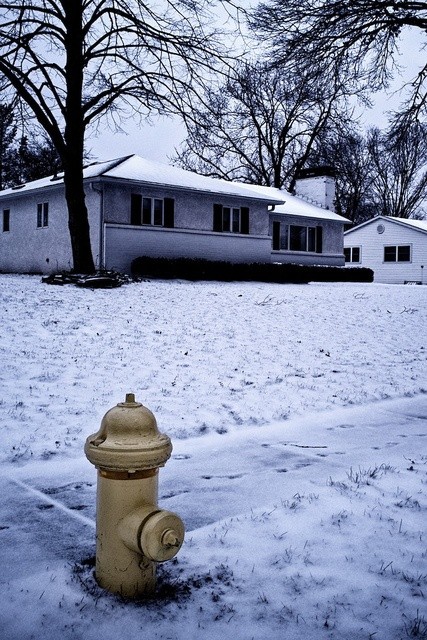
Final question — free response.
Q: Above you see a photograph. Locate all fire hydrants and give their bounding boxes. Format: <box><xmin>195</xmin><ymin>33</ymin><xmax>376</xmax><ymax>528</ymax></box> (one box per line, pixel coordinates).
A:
<box><xmin>85</xmin><ymin>393</ymin><xmax>184</xmax><ymax>594</ymax></box>
